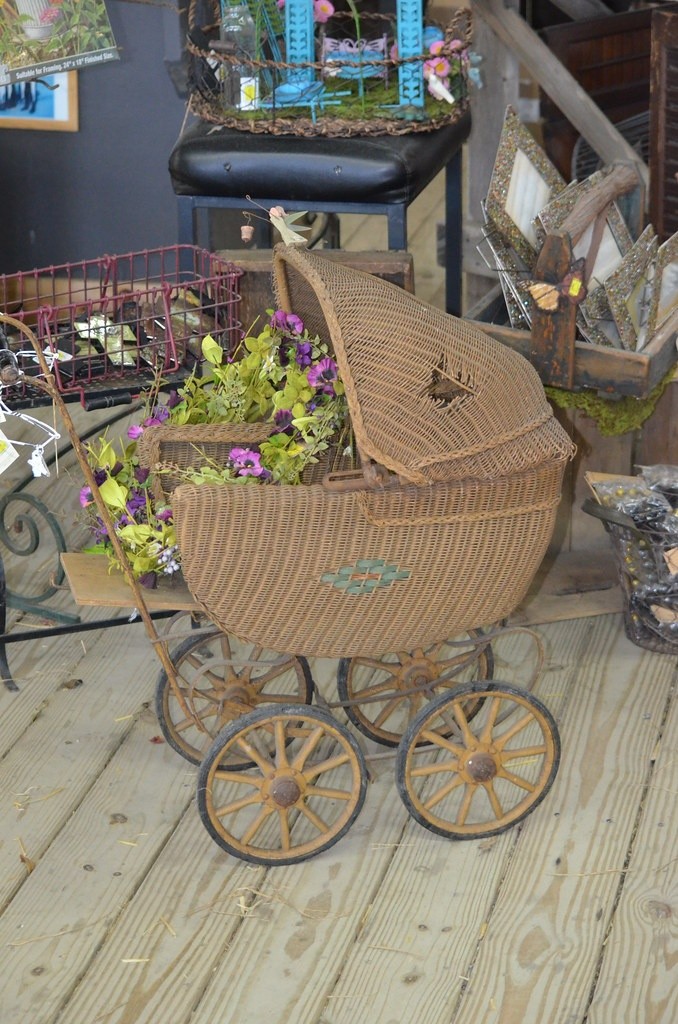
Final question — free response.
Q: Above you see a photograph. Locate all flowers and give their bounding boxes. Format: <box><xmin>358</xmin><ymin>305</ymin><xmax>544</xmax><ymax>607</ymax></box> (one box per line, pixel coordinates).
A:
<box><xmin>77</xmin><ymin>306</ymin><xmax>347</xmax><ymax>590</ymax></box>
<box><xmin>392</xmin><ymin>39</ymin><xmax>474</xmax><ymax>102</ymax></box>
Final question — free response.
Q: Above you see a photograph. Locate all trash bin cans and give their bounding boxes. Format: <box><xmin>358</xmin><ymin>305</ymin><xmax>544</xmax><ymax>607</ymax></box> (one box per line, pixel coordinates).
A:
<box><xmin>580</xmin><ymin>498</ymin><xmax>678</xmax><ymax>656</ymax></box>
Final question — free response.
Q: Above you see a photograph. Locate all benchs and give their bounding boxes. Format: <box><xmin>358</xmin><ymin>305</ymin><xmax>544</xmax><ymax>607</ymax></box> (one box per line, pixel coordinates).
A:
<box><xmin>321</xmin><ymin>33</ymin><xmax>390</xmax><ymax>90</ymax></box>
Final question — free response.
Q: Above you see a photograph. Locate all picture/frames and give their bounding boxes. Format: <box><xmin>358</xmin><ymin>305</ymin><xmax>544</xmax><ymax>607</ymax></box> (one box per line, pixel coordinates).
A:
<box><xmin>1</xmin><ymin>69</ymin><xmax>79</xmax><ymax>133</ymax></box>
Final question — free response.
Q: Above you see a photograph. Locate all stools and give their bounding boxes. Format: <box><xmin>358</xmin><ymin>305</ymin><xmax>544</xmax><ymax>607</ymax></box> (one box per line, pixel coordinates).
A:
<box><xmin>167</xmin><ymin>118</ymin><xmax>464</xmax><ymax>322</ymax></box>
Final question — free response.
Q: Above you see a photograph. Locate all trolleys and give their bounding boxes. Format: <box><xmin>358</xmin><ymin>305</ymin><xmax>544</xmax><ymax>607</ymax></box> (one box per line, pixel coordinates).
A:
<box><xmin>0</xmin><ymin>239</ymin><xmax>576</xmax><ymax>869</ymax></box>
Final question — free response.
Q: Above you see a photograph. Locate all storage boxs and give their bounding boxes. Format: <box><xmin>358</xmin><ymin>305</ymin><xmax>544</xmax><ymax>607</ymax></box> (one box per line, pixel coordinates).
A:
<box><xmin>187</xmin><ymin>1</ymin><xmax>473</xmax><ymax>137</ymax></box>
<box><xmin>582</xmin><ymin>497</ymin><xmax>678</xmax><ymax>656</ymax></box>
<box><xmin>459</xmin><ymin>157</ymin><xmax>677</xmax><ymax>398</ymax></box>
